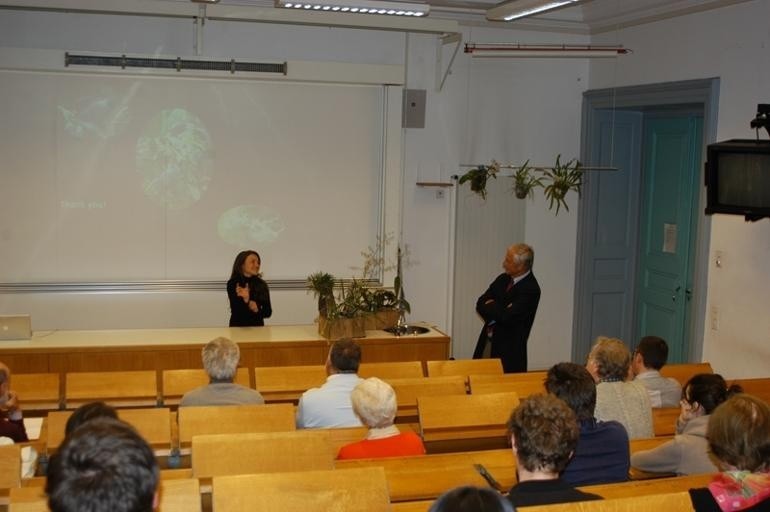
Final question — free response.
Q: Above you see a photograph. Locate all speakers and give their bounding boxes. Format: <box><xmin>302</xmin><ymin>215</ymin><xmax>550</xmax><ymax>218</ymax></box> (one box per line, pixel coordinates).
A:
<box><xmin>402</xmin><ymin>89</ymin><xmax>426</xmax><ymax>128</ymax></box>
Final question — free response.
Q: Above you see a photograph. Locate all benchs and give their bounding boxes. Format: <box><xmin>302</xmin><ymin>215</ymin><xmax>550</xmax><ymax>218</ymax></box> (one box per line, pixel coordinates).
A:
<box><xmin>1</xmin><ymin>359</ymin><xmax>770</xmax><ymax>512</ymax></box>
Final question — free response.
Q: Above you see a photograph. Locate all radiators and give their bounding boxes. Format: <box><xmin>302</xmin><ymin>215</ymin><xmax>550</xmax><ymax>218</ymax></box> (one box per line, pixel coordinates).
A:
<box><xmin>446</xmin><ymin>174</ymin><xmax>527</xmax><ymax>360</ymax></box>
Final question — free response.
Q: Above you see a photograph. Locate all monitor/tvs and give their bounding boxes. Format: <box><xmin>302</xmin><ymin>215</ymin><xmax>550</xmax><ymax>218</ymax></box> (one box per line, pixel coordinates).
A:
<box><xmin>704</xmin><ymin>139</ymin><xmax>770</xmax><ymax>222</ymax></box>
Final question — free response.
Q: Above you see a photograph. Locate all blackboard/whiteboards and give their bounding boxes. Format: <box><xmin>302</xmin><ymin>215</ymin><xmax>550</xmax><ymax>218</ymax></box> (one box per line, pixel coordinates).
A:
<box><xmin>0</xmin><ymin>69</ymin><xmax>386</xmax><ymax>291</ymax></box>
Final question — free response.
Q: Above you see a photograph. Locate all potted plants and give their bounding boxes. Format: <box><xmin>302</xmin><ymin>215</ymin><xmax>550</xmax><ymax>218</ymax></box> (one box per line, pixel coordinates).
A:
<box><xmin>346</xmin><ymin>275</ymin><xmax>410</xmax><ymax>331</ymax></box>
<box><xmin>506</xmin><ymin>158</ymin><xmax>553</xmax><ymax>199</ymax></box>
<box><xmin>303</xmin><ymin>270</ymin><xmax>377</xmax><ymax>339</ymax></box>
<box><xmin>458</xmin><ymin>158</ymin><xmax>502</xmax><ymax>200</ymax></box>
<box><xmin>534</xmin><ymin>154</ymin><xmax>585</xmax><ymax>216</ymax></box>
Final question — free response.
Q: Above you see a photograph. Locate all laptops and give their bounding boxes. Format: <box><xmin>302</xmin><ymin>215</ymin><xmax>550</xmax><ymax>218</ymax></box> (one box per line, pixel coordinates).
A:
<box><xmin>0</xmin><ymin>314</ymin><xmax>32</xmax><ymax>340</ymax></box>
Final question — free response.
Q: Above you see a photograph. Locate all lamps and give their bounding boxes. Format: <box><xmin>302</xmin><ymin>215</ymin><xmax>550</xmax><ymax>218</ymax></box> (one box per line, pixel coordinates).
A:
<box><xmin>463</xmin><ymin>0</ymin><xmax>633</xmax><ymax>58</ymax></box>
<box><xmin>485</xmin><ymin>0</ymin><xmax>594</xmax><ymax>22</ymax></box>
<box><xmin>274</xmin><ymin>0</ymin><xmax>430</xmax><ymax>18</ymax></box>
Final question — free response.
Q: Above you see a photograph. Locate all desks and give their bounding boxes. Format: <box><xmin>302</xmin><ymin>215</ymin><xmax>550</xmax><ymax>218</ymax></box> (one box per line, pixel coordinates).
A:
<box><xmin>0</xmin><ymin>321</ymin><xmax>451</xmax><ymax>410</ymax></box>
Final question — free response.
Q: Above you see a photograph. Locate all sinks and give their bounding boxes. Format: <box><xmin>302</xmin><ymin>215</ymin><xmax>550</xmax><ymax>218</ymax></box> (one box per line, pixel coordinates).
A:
<box><xmin>384</xmin><ymin>325</ymin><xmax>428</xmax><ymax>336</ymax></box>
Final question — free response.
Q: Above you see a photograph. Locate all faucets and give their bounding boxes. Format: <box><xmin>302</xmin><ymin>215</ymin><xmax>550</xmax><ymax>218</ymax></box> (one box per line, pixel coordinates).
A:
<box><xmin>397</xmin><ymin>319</ymin><xmax>401</xmax><ymax>327</ymax></box>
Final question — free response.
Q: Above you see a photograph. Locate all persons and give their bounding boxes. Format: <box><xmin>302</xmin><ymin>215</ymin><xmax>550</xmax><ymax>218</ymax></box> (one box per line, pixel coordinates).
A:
<box><xmin>65</xmin><ymin>402</ymin><xmax>117</xmax><ymax>434</ymax></box>
<box><xmin>336</xmin><ymin>376</ymin><xmax>427</xmax><ymax>462</ymax></box>
<box><xmin>296</xmin><ymin>337</ymin><xmax>367</xmax><ymax>428</ymax></box>
<box><xmin>586</xmin><ymin>336</ymin><xmax>656</xmax><ymax>441</ymax></box>
<box><xmin>544</xmin><ymin>362</ymin><xmax>631</xmax><ymax>486</ymax></box>
<box><xmin>472</xmin><ymin>243</ymin><xmax>541</xmax><ymax>373</ymax></box>
<box><xmin>632</xmin><ymin>373</ymin><xmax>744</xmax><ymax>477</ymax></box>
<box><xmin>426</xmin><ymin>487</ymin><xmax>515</xmax><ymax>511</ymax></box>
<box><xmin>633</xmin><ymin>337</ymin><xmax>682</xmax><ymax>408</ymax></box>
<box><xmin>45</xmin><ymin>417</ymin><xmax>163</xmax><ymax>511</ymax></box>
<box><xmin>688</xmin><ymin>393</ymin><xmax>770</xmax><ymax>511</ymax></box>
<box><xmin>179</xmin><ymin>337</ymin><xmax>266</xmax><ymax>405</ymax></box>
<box><xmin>504</xmin><ymin>392</ymin><xmax>602</xmax><ymax>507</ymax></box>
<box><xmin>0</xmin><ymin>362</ymin><xmax>28</xmax><ymax>442</ymax></box>
<box><xmin>227</xmin><ymin>250</ymin><xmax>272</xmax><ymax>326</ymax></box>
